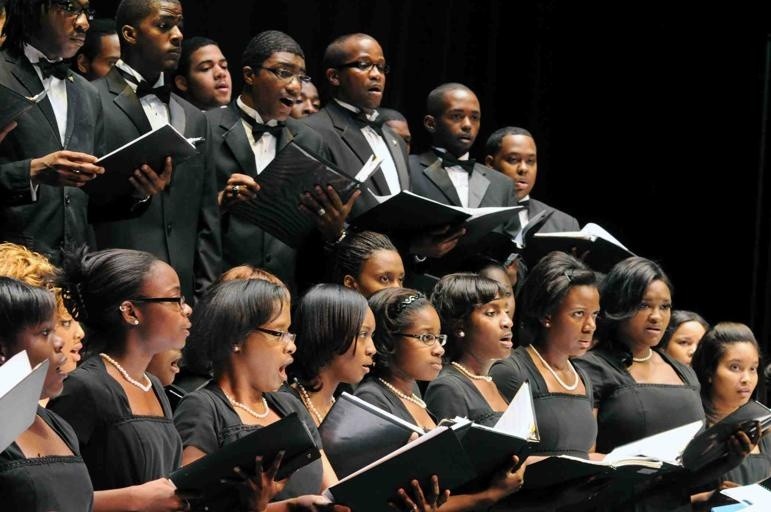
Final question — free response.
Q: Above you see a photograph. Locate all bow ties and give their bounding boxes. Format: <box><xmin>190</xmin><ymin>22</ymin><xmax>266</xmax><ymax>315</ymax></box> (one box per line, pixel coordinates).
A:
<box><xmin>517</xmin><ymin>198</ymin><xmax>531</xmax><ymax>206</ymax></box>
<box><xmin>38</xmin><ymin>55</ymin><xmax>71</xmax><ymax>81</ymax></box>
<box><xmin>136</xmin><ymin>79</ymin><xmax>171</xmax><ymax>104</ymax></box>
<box><xmin>441</xmin><ymin>150</ymin><xmax>476</xmax><ymax>175</ymax></box>
<box><xmin>251</xmin><ymin>120</ymin><xmax>283</xmax><ymax>142</ymax></box>
<box><xmin>355</xmin><ymin>110</ymin><xmax>383</xmax><ymax>136</ymax></box>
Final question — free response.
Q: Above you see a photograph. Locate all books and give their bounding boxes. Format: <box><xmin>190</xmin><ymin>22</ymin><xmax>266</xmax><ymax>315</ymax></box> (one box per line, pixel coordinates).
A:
<box><xmin>435</xmin><ymin>376</ymin><xmax>541</xmax><ymax>495</ymax></box>
<box><xmin>528</xmin><ymin>220</ymin><xmax>639</xmax><ymax>270</ymax></box>
<box><xmin>318</xmin><ymin>392</ymin><xmax>429</xmax><ymax>481</ymax></box>
<box><xmin>524</xmin><ymin>417</ymin><xmax>707</xmax><ymax>510</ymax></box>
<box><xmin>323</xmin><ymin>425</ymin><xmax>479</xmax><ymax>512</ymax></box>
<box><xmin>0</xmin><ymin>80</ymin><xmax>48</xmax><ymax>137</ymax></box>
<box><xmin>224</xmin><ymin>137</ymin><xmax>387</xmax><ymax>257</ymax></box>
<box><xmin>78</xmin><ymin>121</ymin><xmax>207</xmax><ymax>220</ymax></box>
<box><xmin>461</xmin><ymin>208</ymin><xmax>556</xmax><ymax>270</ymax></box>
<box><xmin>349</xmin><ymin>188</ymin><xmax>524</xmax><ymax>261</ymax></box>
<box><xmin>682</xmin><ymin>400</ymin><xmax>771</xmax><ymax>477</ymax></box>
<box><xmin>165</xmin><ymin>410</ymin><xmax>321</xmax><ymax>511</ymax></box>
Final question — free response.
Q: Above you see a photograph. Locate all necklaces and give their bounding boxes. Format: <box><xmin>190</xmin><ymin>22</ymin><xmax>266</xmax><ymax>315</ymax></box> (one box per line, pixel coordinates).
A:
<box><xmin>527</xmin><ymin>343</ymin><xmax>579</xmax><ymax>391</ymax></box>
<box><xmin>451</xmin><ymin>360</ymin><xmax>495</xmax><ymax>384</ymax></box>
<box><xmin>96</xmin><ymin>351</ymin><xmax>154</xmax><ymax>394</ymax></box>
<box><xmin>621</xmin><ymin>349</ymin><xmax>655</xmax><ymax>364</ymax></box>
<box><xmin>376</xmin><ymin>375</ymin><xmax>427</xmax><ymax>410</ymax></box>
<box><xmin>293</xmin><ymin>375</ymin><xmax>337</xmax><ymax>427</ymax></box>
<box><xmin>217</xmin><ymin>384</ymin><xmax>271</xmax><ymax>420</ymax></box>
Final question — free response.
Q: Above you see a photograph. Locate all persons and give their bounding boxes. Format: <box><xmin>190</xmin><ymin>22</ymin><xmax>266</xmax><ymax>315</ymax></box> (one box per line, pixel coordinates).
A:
<box><xmin>277</xmin><ymin>283</ymin><xmax>451</xmax><ymax>512</ymax></box>
<box><xmin>570</xmin><ymin>256</ymin><xmax>771</xmax><ymax>512</ymax></box>
<box><xmin>416</xmin><ymin>272</ymin><xmax>527</xmax><ymax>511</ymax></box>
<box><xmin>376</xmin><ymin>108</ymin><xmax>411</xmax><ymax>156</ymax></box>
<box><xmin>63</xmin><ymin>20</ymin><xmax>120</xmax><ymax>82</ymax></box>
<box><xmin>90</xmin><ymin>0</ymin><xmax>221</xmax><ymax>310</ymax></box>
<box><xmin>653</xmin><ymin>309</ymin><xmax>710</xmax><ymax>368</ymax></box>
<box><xmin>0</xmin><ymin>0</ymin><xmax>173</xmax><ymax>269</ymax></box>
<box><xmin>301</xmin><ymin>33</ymin><xmax>459</xmax><ymax>288</ymax></box>
<box><xmin>487</xmin><ymin>249</ymin><xmax>611</xmax><ymax>512</ymax></box>
<box><xmin>0</xmin><ymin>242</ymin><xmax>85</xmax><ymax>376</ymax></box>
<box><xmin>43</xmin><ymin>244</ymin><xmax>296</xmax><ymax>512</ymax></box>
<box><xmin>690</xmin><ymin>322</ymin><xmax>771</xmax><ymax>512</ymax></box>
<box><xmin>144</xmin><ymin>346</ymin><xmax>184</xmax><ymax>391</ymax></box>
<box><xmin>456</xmin><ymin>258</ymin><xmax>515</xmax><ymax>325</ymax></box>
<box><xmin>193</xmin><ymin>29</ymin><xmax>362</xmax><ymax>304</ymax></box>
<box><xmin>485</xmin><ymin>127</ymin><xmax>600</xmax><ymax>272</ymax></box>
<box><xmin>172</xmin><ymin>277</ymin><xmax>350</xmax><ymax>512</ymax></box>
<box><xmin>167</xmin><ymin>37</ymin><xmax>232</xmax><ymax>113</ymax></box>
<box><xmin>327</xmin><ymin>231</ymin><xmax>405</xmax><ymax>300</ymax></box>
<box><xmin>349</xmin><ymin>287</ymin><xmax>527</xmax><ymax>511</ymax></box>
<box><xmin>404</xmin><ymin>81</ymin><xmax>522</xmax><ymax>299</ymax></box>
<box><xmin>289</xmin><ymin>77</ymin><xmax>321</xmax><ymax>120</ymax></box>
<box><xmin>0</xmin><ymin>275</ymin><xmax>94</xmax><ymax>511</ymax></box>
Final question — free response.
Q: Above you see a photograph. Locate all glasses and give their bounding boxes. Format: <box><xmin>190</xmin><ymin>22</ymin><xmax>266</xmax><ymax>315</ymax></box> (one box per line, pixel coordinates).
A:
<box><xmin>342</xmin><ymin>59</ymin><xmax>390</xmax><ymax>75</ymax></box>
<box><xmin>133</xmin><ymin>294</ymin><xmax>187</xmax><ymax>310</ymax></box>
<box><xmin>57</xmin><ymin>0</ymin><xmax>95</xmax><ymax>21</ymax></box>
<box><xmin>397</xmin><ymin>332</ymin><xmax>447</xmax><ymax>347</ymax></box>
<box><xmin>260</xmin><ymin>66</ymin><xmax>311</xmax><ymax>86</ymax></box>
<box><xmin>255</xmin><ymin>327</ymin><xmax>297</xmax><ymax>345</ymax></box>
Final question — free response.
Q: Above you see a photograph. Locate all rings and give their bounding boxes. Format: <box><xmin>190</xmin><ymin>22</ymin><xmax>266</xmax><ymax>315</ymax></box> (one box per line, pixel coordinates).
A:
<box><xmin>230</xmin><ymin>186</ymin><xmax>241</xmax><ymax>195</ymax></box>
<box><xmin>316</xmin><ymin>208</ymin><xmax>326</xmax><ymax>219</ymax></box>
